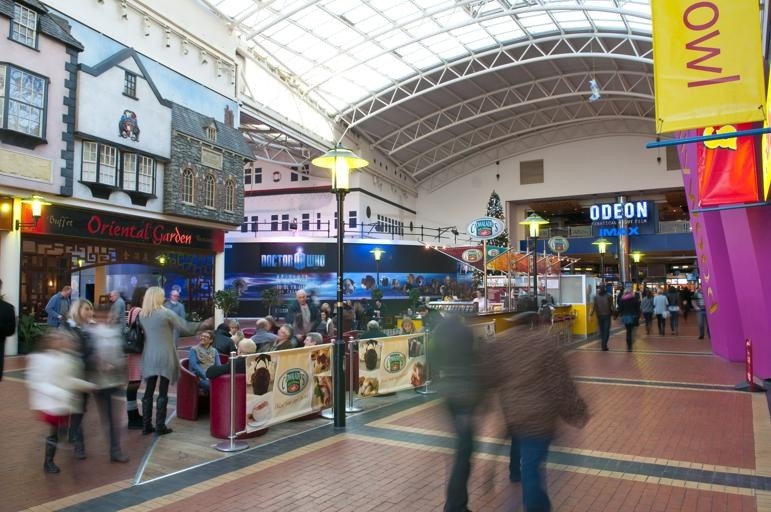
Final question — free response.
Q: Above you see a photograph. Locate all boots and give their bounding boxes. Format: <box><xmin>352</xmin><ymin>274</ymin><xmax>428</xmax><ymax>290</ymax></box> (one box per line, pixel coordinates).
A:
<box><xmin>142</xmin><ymin>398</ymin><xmax>155</xmax><ymax>434</ymax></box>
<box><xmin>156</xmin><ymin>397</ymin><xmax>173</xmax><ymax>435</ymax></box>
<box><xmin>127</xmin><ymin>408</ymin><xmax>142</xmax><ymax>429</ymax></box>
<box><xmin>43</xmin><ymin>436</ymin><xmax>60</xmax><ymax>473</ymax></box>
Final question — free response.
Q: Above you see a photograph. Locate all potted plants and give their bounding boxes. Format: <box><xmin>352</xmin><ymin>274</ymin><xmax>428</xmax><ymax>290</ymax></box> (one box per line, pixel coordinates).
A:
<box><xmin>17</xmin><ymin>315</ymin><xmax>41</xmax><ymax>353</ymax></box>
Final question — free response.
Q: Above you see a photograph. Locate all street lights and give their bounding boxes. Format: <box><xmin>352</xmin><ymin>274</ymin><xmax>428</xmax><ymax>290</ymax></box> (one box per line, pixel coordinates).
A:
<box><xmin>518</xmin><ymin>211</ymin><xmax>551</xmax><ymax>312</ymax></box>
<box><xmin>369</xmin><ymin>247</ymin><xmax>387</xmax><ymax>289</ymax></box>
<box><xmin>627</xmin><ymin>249</ymin><xmax>647</xmax><ymax>285</ymax></box>
<box><xmin>591</xmin><ymin>237</ymin><xmax>614</xmax><ymax>283</ymax></box>
<box><xmin>78</xmin><ymin>258</ymin><xmax>83</xmax><ymax>298</ymax></box>
<box><xmin>154</xmin><ymin>254</ymin><xmax>171</xmax><ymax>289</ymax></box>
<box><xmin>310</xmin><ymin>143</ymin><xmax>372</xmax><ymax>427</ymax></box>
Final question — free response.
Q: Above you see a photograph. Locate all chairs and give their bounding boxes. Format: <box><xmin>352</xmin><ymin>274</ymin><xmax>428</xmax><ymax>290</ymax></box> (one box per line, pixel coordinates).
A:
<box><xmin>289</xmin><ymin>409</ymin><xmax>322</xmax><ymax>420</ymax></box>
<box><xmin>323</xmin><ymin>329</ymin><xmax>359</xmax><ymax>394</ymax></box>
<box><xmin>177</xmin><ymin>353</ymin><xmax>230</xmax><ymax>421</ymax></box>
<box><xmin>241</xmin><ymin>327</ymin><xmax>256</xmax><ymax>338</ymax></box>
<box><xmin>210</xmin><ymin>372</ymin><xmax>267</xmax><ymax>438</ymax></box>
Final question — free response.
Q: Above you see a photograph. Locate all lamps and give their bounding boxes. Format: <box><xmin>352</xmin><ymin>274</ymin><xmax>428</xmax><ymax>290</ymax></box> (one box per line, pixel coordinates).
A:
<box><xmin>16</xmin><ymin>195</ymin><xmax>52</xmax><ymax>230</ymax></box>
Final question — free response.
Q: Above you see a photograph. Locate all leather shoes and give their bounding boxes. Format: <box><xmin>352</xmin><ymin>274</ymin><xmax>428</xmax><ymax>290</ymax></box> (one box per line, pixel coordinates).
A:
<box><xmin>75</xmin><ymin>445</ymin><xmax>86</xmax><ymax>459</ymax></box>
<box><xmin>111</xmin><ymin>446</ymin><xmax>129</xmax><ymax>462</ymax></box>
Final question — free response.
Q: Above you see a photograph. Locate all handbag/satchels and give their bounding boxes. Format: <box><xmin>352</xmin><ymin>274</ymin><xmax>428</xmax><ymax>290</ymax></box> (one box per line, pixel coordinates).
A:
<box><xmin>122</xmin><ymin>315</ymin><xmax>146</xmax><ymax>355</ymax></box>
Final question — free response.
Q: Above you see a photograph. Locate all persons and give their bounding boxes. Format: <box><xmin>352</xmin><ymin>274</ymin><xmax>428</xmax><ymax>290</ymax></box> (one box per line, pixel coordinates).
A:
<box><xmin>589</xmin><ymin>287</ymin><xmax>616</xmax><ymax>351</ymax></box>
<box><xmin>189</xmin><ymin>289</ymin><xmax>441</xmax><ymax>397</ymax></box>
<box><xmin>614</xmin><ymin>289</ymin><xmax>641</xmax><ymax>352</ymax></box>
<box><xmin>0</xmin><ymin>286</ymin><xmax>16</xmax><ymax>383</ymax></box>
<box><xmin>639</xmin><ymin>287</ymin><xmax>711</xmax><ymax>340</ymax></box>
<box><xmin>485</xmin><ymin>296</ymin><xmax>590</xmax><ymax>512</ymax></box>
<box><xmin>472</xmin><ymin>289</ymin><xmax>489</xmax><ymax>311</ymax></box>
<box><xmin>426</xmin><ymin>308</ymin><xmax>494</xmax><ymax>512</ymax></box>
<box><xmin>25</xmin><ymin>286</ymin><xmax>215</xmax><ymax>474</ymax></box>
